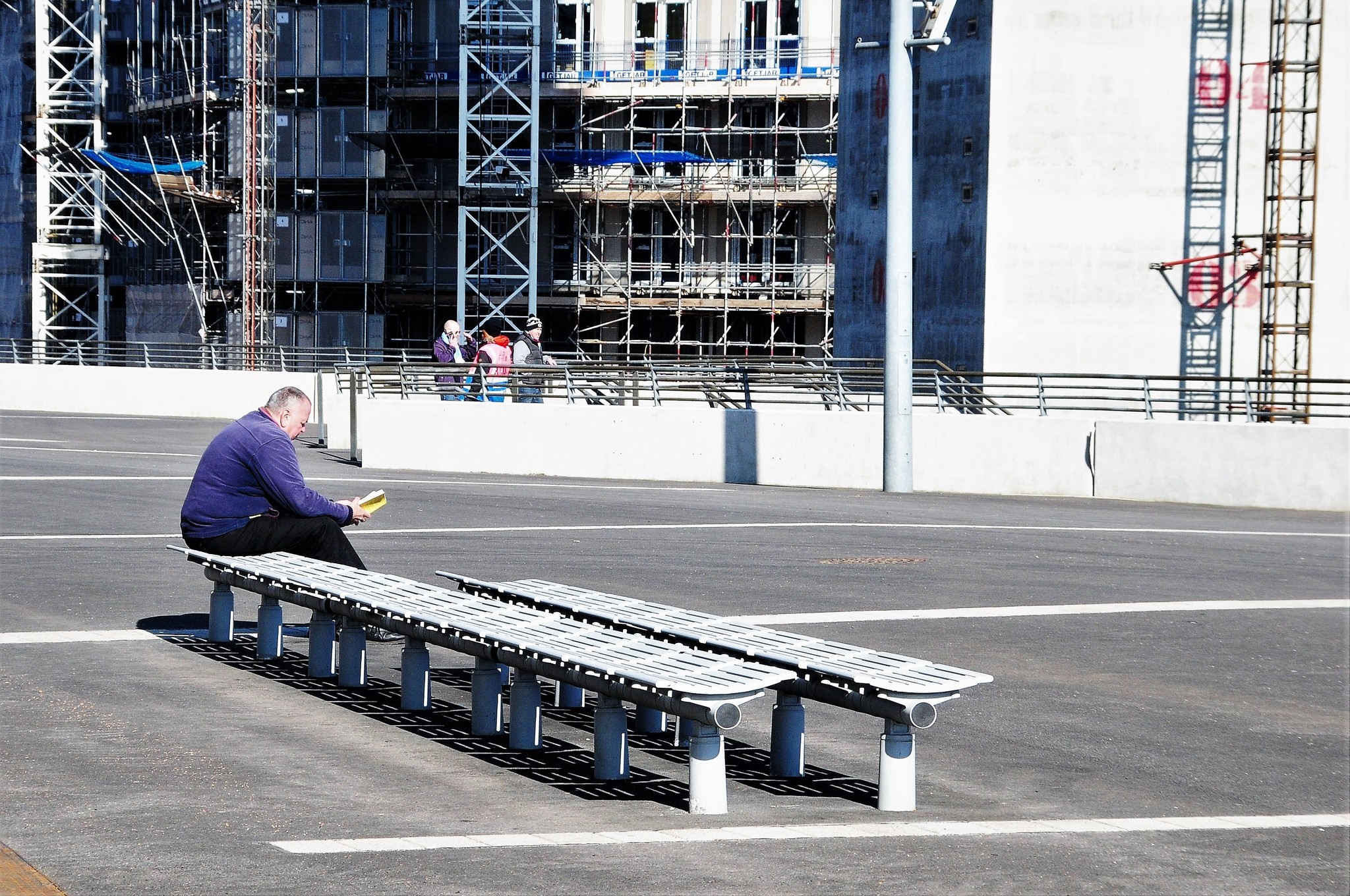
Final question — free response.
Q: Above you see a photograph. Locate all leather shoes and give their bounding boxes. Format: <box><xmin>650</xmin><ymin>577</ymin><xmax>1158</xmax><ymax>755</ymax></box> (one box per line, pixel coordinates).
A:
<box><xmin>307</xmin><ymin>624</ymin><xmax>342</xmax><ymax>642</ymax></box>
<box><xmin>366</xmin><ymin>626</ymin><xmax>405</xmax><ymax>645</ymax></box>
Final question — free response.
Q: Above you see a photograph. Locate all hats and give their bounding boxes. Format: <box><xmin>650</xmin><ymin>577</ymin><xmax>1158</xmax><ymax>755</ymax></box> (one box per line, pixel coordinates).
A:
<box><xmin>524</xmin><ymin>314</ymin><xmax>542</xmax><ymax>331</ymax></box>
<box><xmin>482</xmin><ymin>317</ymin><xmax>501</xmax><ymax>338</ymax></box>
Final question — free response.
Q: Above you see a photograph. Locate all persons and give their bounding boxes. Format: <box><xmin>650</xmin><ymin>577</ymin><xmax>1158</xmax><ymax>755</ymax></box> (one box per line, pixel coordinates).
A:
<box><xmin>464</xmin><ymin>320</ymin><xmax>512</xmax><ymax>402</ymax></box>
<box><xmin>512</xmin><ymin>313</ymin><xmax>557</xmax><ymax>404</ymax></box>
<box><xmin>180</xmin><ymin>387</ymin><xmax>405</xmax><ymax>645</ymax></box>
<box><xmin>431</xmin><ymin>320</ymin><xmax>477</xmax><ymax>401</ymax></box>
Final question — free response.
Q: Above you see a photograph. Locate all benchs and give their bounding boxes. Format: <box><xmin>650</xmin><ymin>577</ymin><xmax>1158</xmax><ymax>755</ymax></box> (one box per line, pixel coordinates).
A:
<box><xmin>165</xmin><ymin>548</ymin><xmax>994</xmax><ymax>813</ymax></box>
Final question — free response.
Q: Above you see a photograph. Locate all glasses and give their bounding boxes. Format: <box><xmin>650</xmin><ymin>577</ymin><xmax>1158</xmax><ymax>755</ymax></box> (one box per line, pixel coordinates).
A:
<box><xmin>448</xmin><ymin>329</ymin><xmax>460</xmax><ymax>334</ymax></box>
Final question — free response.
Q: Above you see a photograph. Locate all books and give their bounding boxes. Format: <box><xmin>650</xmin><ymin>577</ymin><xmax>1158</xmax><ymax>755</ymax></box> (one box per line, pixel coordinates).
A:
<box><xmin>335</xmin><ymin>490</ymin><xmax>387</xmax><ymax>514</ymax></box>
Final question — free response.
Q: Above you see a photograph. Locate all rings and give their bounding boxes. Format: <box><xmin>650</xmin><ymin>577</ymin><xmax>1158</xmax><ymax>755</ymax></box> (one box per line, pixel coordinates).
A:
<box><xmin>355</xmin><ymin>522</ymin><xmax>359</xmax><ymax>524</ymax></box>
